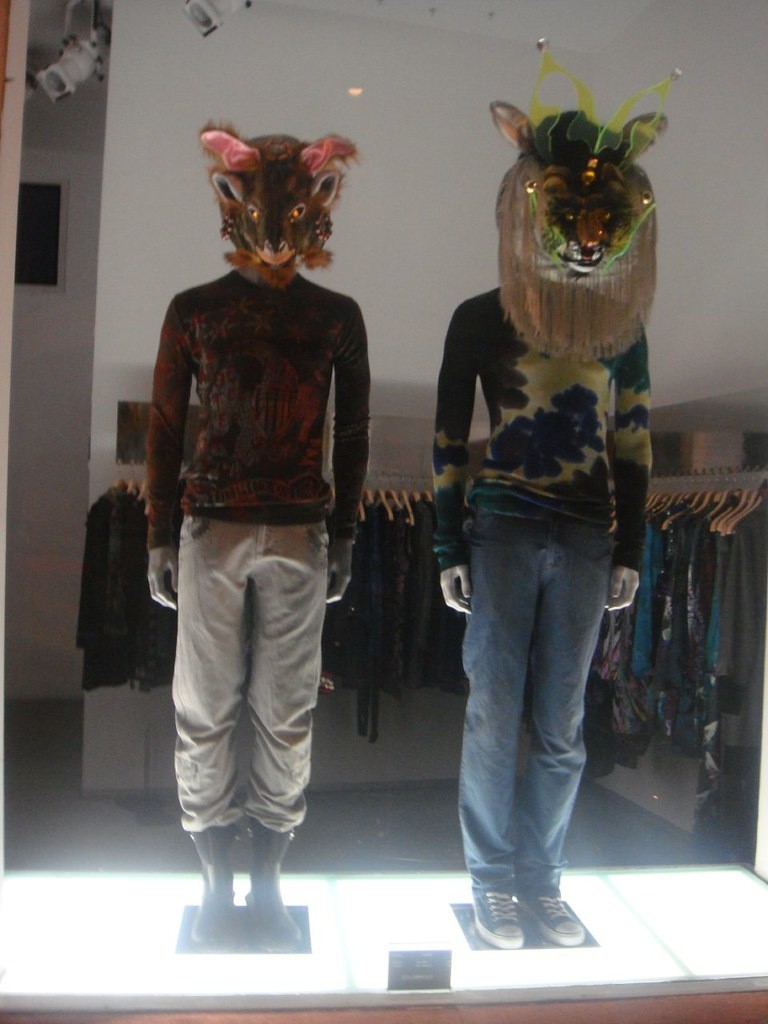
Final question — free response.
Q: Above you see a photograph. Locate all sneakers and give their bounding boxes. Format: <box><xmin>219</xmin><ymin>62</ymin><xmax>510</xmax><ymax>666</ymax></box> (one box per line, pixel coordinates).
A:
<box><xmin>473</xmin><ymin>883</ymin><xmax>525</xmax><ymax>949</ymax></box>
<box><xmin>517</xmin><ymin>887</ymin><xmax>585</xmax><ymax>947</ymax></box>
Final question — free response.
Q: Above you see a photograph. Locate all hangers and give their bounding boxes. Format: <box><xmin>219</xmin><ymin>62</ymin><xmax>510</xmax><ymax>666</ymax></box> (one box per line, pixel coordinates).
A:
<box><xmin>102</xmin><ymin>453</ymin><xmax>768</xmax><ymax>538</ymax></box>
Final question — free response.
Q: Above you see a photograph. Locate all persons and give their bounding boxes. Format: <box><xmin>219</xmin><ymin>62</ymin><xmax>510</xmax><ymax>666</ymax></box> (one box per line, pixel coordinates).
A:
<box><xmin>144</xmin><ymin>123</ymin><xmax>373</xmax><ymax>950</ymax></box>
<box><xmin>431</xmin><ymin>105</ymin><xmax>666</xmax><ymax>945</ymax></box>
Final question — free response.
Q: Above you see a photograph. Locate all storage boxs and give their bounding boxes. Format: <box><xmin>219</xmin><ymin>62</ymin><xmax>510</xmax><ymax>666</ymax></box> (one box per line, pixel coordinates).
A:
<box><xmin>647</xmin><ymin>430</ymin><xmax>745</xmax><ymax>475</ymax></box>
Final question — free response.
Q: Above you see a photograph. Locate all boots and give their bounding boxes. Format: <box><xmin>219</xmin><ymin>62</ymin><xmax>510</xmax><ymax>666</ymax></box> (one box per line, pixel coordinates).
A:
<box><xmin>191</xmin><ymin>818</ymin><xmax>236</xmax><ymax>944</ymax></box>
<box><xmin>245</xmin><ymin>817</ymin><xmax>302</xmax><ymax>944</ymax></box>
